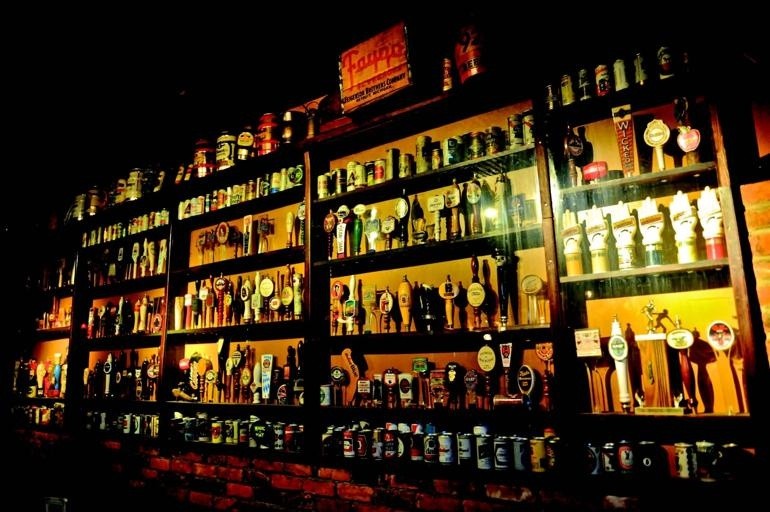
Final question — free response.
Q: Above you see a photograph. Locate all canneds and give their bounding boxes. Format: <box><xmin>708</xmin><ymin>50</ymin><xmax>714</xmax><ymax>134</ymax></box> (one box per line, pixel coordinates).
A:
<box><xmin>586</xmin><ymin>439</ymin><xmax>741</xmax><ymax>485</ymax></box>
<box><xmin>343</xmin><ymin>423</ymin><xmax>560</xmax><ymax>473</ymax></box>
<box><xmin>319</xmin><ymin>385</ymin><xmax>330</xmax><ymax>406</ymax></box>
<box><xmin>84</xmin><ymin>412</ymin><xmax>159</xmax><ymax>438</ymax></box>
<box><xmin>171</xmin><ymin>411</ymin><xmax>304</xmax><ymax>450</ymax></box>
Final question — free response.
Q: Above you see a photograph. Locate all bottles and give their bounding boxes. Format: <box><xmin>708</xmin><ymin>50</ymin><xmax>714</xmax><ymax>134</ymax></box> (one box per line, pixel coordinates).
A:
<box><xmin>25</xmin><ymin>353</ymin><xmax>68</xmax><ymax>398</ymax></box>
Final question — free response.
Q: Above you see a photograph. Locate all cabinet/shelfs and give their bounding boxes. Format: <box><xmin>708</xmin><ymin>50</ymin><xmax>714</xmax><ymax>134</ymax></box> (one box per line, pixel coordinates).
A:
<box><xmin>6</xmin><ymin>22</ymin><xmax>770</xmax><ymax>440</ymax></box>
